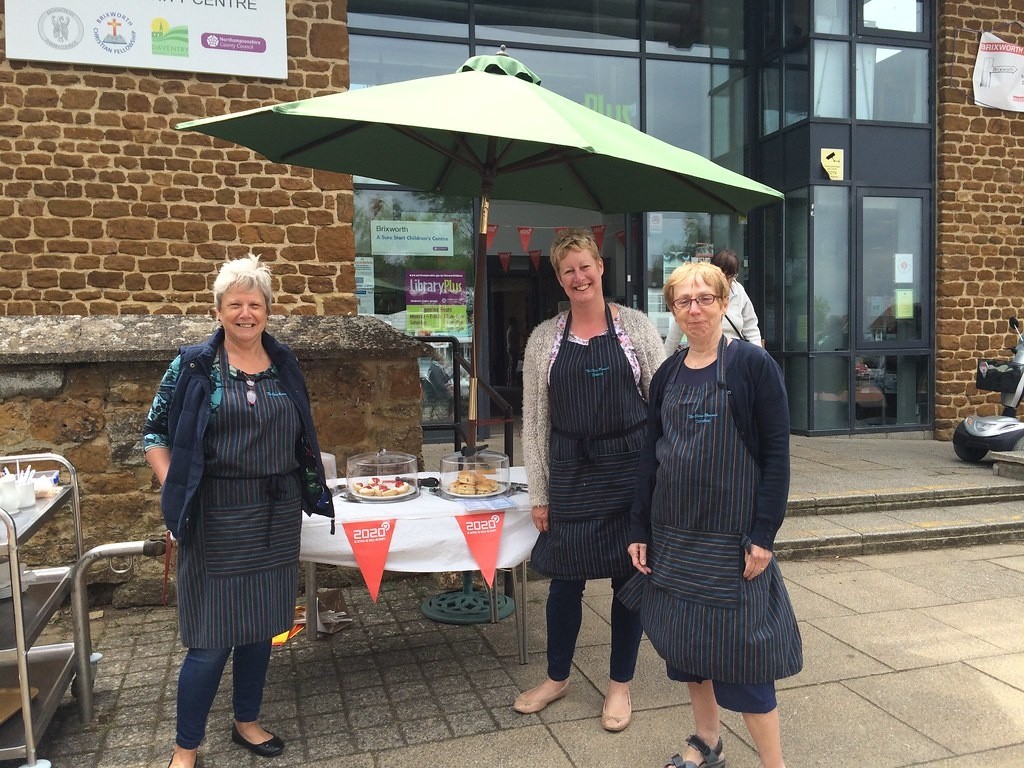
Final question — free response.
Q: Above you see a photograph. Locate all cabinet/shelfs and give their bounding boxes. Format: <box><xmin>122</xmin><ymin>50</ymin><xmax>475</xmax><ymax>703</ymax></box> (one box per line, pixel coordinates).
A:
<box><xmin>0</xmin><ymin>454</ymin><xmax>103</xmax><ymax>768</ymax></box>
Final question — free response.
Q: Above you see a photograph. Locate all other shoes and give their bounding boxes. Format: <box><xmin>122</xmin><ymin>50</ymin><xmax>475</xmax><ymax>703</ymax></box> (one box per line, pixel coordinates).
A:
<box><xmin>168</xmin><ymin>752</ymin><xmax>199</xmax><ymax>768</ymax></box>
<box><xmin>514</xmin><ymin>679</ymin><xmax>569</xmax><ymax>714</ymax></box>
<box><xmin>601</xmin><ymin>689</ymin><xmax>632</xmax><ymax>731</ymax></box>
<box><xmin>232</xmin><ymin>722</ymin><xmax>284</xmax><ymax>757</ymax></box>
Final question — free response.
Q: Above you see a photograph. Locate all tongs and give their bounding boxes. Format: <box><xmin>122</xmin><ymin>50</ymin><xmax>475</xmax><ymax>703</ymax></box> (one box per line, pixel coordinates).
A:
<box><xmin>395</xmin><ymin>476</ymin><xmax>438</xmax><ymax>488</ymax></box>
<box><xmin>461</xmin><ymin>444</ymin><xmax>489</xmax><ymax>457</ymax></box>
<box><xmin>497</xmin><ymin>481</ymin><xmax>528</xmax><ymax>497</ymax></box>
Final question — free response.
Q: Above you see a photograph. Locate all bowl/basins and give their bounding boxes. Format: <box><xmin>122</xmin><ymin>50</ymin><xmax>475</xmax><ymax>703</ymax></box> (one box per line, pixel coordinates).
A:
<box><xmin>14</xmin><ymin>469</ymin><xmax>60</xmax><ymax>499</ymax></box>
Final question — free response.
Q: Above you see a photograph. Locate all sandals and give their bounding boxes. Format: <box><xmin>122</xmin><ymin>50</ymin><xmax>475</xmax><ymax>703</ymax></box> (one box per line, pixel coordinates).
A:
<box><xmin>664</xmin><ymin>734</ymin><xmax>725</xmax><ymax>768</ymax></box>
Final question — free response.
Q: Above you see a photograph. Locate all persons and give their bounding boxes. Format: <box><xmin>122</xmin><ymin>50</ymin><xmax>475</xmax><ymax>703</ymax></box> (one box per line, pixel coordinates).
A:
<box><xmin>514</xmin><ymin>228</ymin><xmax>669</xmax><ymax>733</ymax></box>
<box><xmin>427</xmin><ymin>352</ymin><xmax>455</xmax><ymax>417</ymax></box>
<box><xmin>813</xmin><ymin>313</ymin><xmax>849</xmax><ymax>394</ymax></box>
<box><xmin>665</xmin><ymin>251</ymin><xmax>762</xmax><ymax>358</ymax></box>
<box><xmin>141</xmin><ymin>258</ymin><xmax>335</xmax><ymax>768</ymax></box>
<box><xmin>507</xmin><ymin>317</ymin><xmax>520</xmax><ymax>386</ymax></box>
<box><xmin>616</xmin><ymin>262</ymin><xmax>802</xmax><ymax>768</ymax></box>
<box><xmin>882</xmin><ymin>303</ymin><xmax>927</xmax><ymax>425</ymax></box>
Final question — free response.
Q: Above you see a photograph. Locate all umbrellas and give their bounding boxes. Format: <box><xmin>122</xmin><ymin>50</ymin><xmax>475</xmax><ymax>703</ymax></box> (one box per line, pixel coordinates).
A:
<box><xmin>449</xmin><ymin>325</ymin><xmax>473</xmax><ymax>337</ymax></box>
<box><xmin>175</xmin><ymin>41</ymin><xmax>785</xmax><ymax>590</ymax></box>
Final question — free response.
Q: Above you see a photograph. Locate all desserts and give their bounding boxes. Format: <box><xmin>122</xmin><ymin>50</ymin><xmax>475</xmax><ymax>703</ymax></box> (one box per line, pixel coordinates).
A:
<box><xmin>352</xmin><ymin>478</ymin><xmax>410</xmax><ymax>496</ymax></box>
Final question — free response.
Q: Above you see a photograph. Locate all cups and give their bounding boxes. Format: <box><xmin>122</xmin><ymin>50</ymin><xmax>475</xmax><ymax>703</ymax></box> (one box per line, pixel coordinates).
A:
<box><xmin>0</xmin><ymin>480</ymin><xmax>20</xmax><ymax>515</ymax></box>
<box><xmin>14</xmin><ymin>479</ymin><xmax>36</xmax><ymax>509</ymax></box>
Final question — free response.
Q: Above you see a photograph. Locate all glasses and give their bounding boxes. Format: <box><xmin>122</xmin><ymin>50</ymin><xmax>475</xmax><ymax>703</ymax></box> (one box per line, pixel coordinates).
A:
<box><xmin>673</xmin><ymin>293</ymin><xmax>726</xmax><ymax>310</ymax></box>
<box><xmin>725</xmin><ymin>272</ymin><xmax>739</xmax><ymax>279</ymax></box>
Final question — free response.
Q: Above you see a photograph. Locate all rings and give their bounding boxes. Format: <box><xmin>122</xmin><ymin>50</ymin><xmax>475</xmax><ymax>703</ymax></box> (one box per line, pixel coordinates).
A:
<box><xmin>760</xmin><ymin>568</ymin><xmax>764</xmax><ymax>571</ymax></box>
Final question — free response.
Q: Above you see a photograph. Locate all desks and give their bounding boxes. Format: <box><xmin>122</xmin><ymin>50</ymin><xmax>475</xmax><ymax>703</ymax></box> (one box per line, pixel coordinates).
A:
<box><xmin>300</xmin><ymin>466</ymin><xmax>540</xmax><ymax>665</ymax></box>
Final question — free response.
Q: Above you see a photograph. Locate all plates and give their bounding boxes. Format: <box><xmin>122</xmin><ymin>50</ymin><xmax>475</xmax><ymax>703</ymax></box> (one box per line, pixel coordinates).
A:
<box><xmin>443</xmin><ymin>482</ymin><xmax>506</xmax><ymax>498</ymax></box>
<box><xmin>350</xmin><ymin>484</ymin><xmax>416</xmax><ymax>501</ymax></box>
<box><xmin>0</xmin><ymin>582</ymin><xmax>28</xmax><ymax>599</ymax></box>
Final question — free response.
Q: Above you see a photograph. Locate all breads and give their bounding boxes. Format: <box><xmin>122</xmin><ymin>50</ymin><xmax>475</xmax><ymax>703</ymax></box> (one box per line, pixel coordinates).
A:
<box><xmin>450</xmin><ymin>471</ymin><xmax>498</xmax><ymax>495</ymax></box>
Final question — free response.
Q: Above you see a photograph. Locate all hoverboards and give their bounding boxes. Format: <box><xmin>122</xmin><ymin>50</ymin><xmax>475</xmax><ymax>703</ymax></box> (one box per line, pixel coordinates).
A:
<box><xmin>952</xmin><ymin>316</ymin><xmax>1024</xmax><ymax>462</ymax></box>
